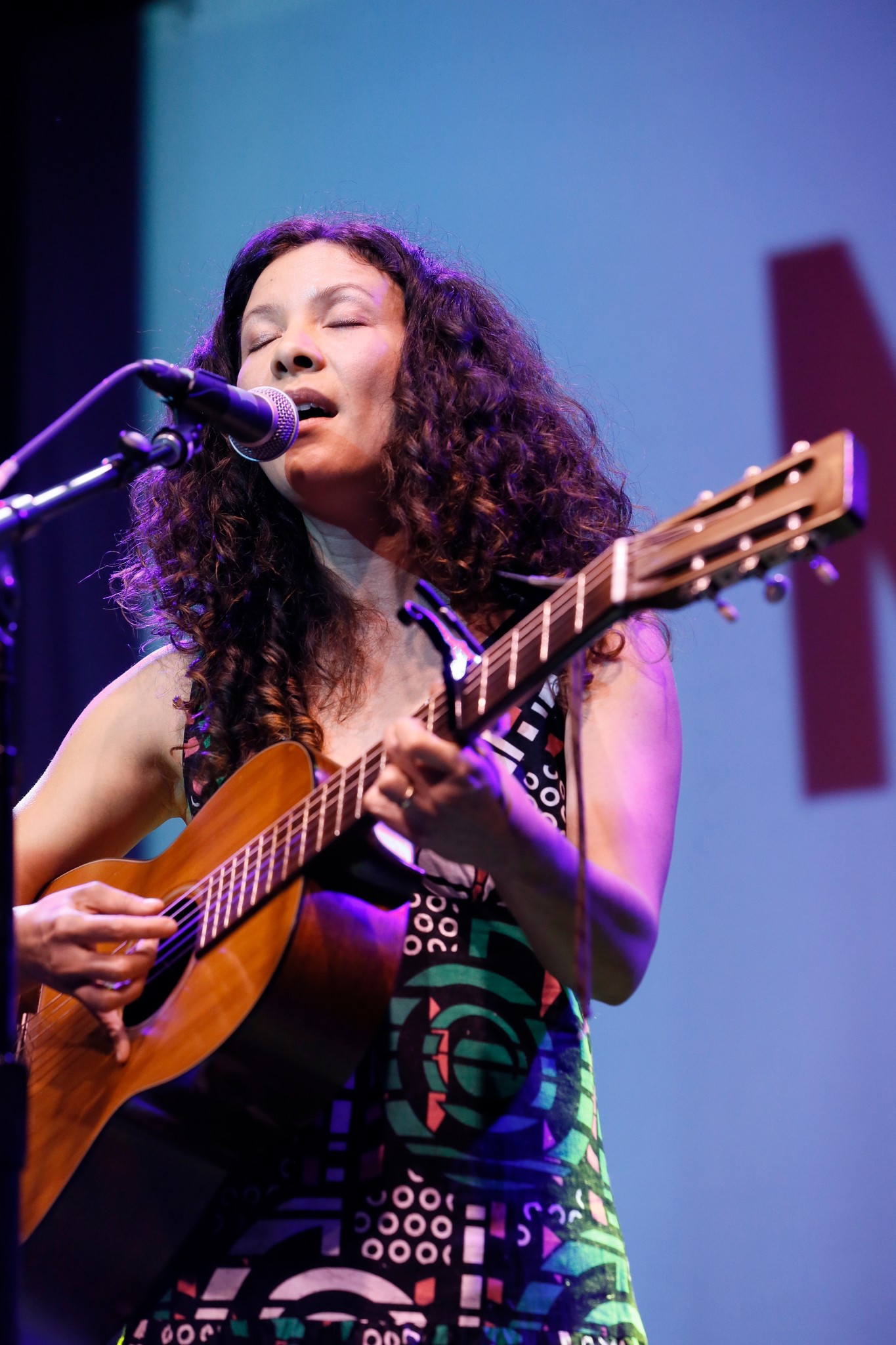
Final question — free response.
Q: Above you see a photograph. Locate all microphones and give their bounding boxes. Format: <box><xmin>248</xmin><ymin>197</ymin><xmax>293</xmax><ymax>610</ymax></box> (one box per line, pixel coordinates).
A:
<box><xmin>137</xmin><ymin>359</ymin><xmax>300</xmax><ymax>463</ymax></box>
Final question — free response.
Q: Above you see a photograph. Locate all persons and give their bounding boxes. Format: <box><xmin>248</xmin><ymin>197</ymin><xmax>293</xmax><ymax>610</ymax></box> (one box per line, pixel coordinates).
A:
<box><xmin>11</xmin><ymin>220</ymin><xmax>680</xmax><ymax>1345</ymax></box>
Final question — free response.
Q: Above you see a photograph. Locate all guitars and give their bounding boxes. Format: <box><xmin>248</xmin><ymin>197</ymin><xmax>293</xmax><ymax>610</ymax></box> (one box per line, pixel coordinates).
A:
<box><xmin>6</xmin><ymin>421</ymin><xmax>870</xmax><ymax>1345</ymax></box>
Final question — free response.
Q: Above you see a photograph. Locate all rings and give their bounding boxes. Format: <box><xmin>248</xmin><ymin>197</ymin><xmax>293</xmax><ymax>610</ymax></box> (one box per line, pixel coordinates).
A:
<box><xmin>400</xmin><ymin>784</ymin><xmax>414</xmax><ymax>811</ymax></box>
